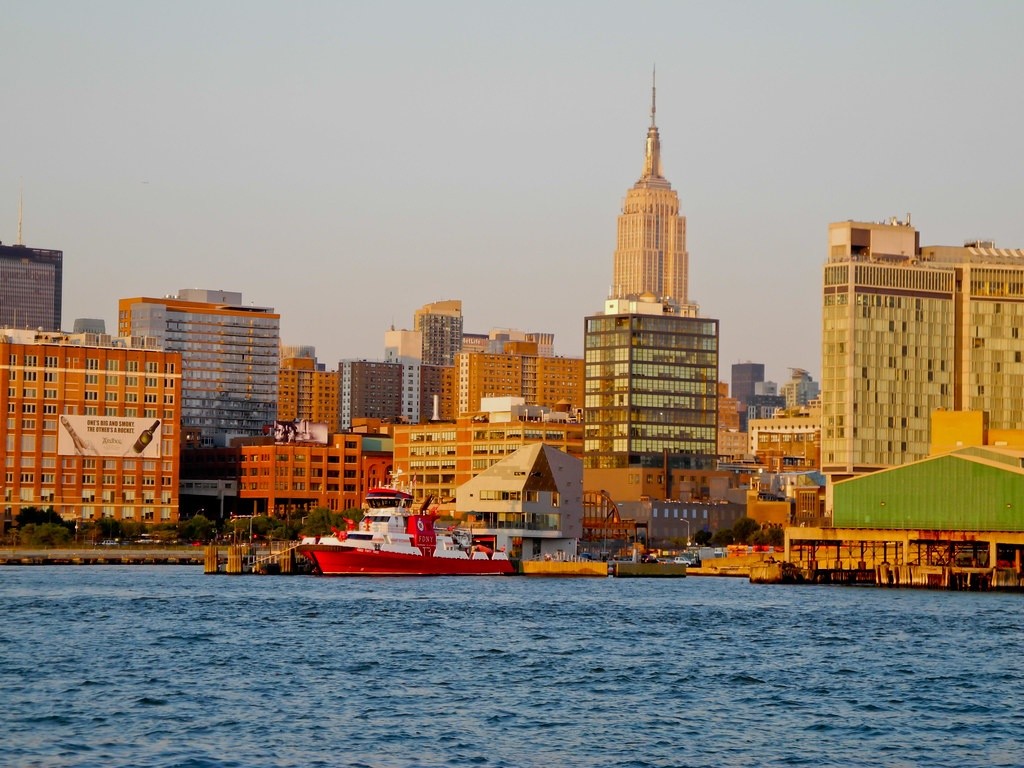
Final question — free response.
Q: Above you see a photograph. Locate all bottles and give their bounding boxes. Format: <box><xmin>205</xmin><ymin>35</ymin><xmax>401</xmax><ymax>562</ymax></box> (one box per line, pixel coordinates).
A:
<box><xmin>60</xmin><ymin>416</ymin><xmax>98</xmax><ymax>455</ymax></box>
<box><xmin>133</xmin><ymin>420</ymin><xmax>160</xmax><ymax>453</ymax></box>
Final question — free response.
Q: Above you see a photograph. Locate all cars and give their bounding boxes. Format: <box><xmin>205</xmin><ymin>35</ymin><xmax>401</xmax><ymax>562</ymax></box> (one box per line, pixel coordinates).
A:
<box><xmin>99</xmin><ymin>533</ymin><xmax>202</xmax><ymax>547</ymax></box>
<box><xmin>640</xmin><ymin>554</ymin><xmax>692</xmax><ymax>568</ymax></box>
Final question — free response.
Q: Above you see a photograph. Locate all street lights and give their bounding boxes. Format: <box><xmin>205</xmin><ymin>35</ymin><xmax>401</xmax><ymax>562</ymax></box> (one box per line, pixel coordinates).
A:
<box><xmin>197</xmin><ymin>509</ymin><xmax>205</xmax><ymax>515</ymax></box>
<box><xmin>681</xmin><ymin>518</ymin><xmax>691</xmax><ymax>546</ymax></box>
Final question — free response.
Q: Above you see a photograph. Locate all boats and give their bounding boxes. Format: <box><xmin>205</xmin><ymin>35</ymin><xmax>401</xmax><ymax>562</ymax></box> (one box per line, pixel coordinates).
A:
<box><xmin>295</xmin><ymin>464</ymin><xmax>517</xmax><ymax>578</ymax></box>
